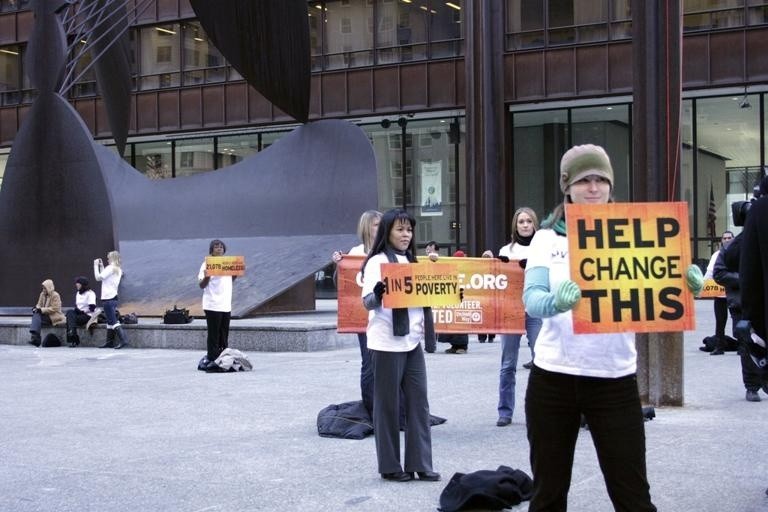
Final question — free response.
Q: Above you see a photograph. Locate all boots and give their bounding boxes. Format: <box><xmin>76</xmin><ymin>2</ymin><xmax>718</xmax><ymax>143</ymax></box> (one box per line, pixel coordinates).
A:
<box><xmin>112</xmin><ymin>322</ymin><xmax>128</xmax><ymax>348</ymax></box>
<box><xmin>99</xmin><ymin>325</ymin><xmax>114</xmax><ymax>347</ymax></box>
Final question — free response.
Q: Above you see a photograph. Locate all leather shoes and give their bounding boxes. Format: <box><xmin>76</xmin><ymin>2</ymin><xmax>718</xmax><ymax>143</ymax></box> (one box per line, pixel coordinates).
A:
<box><xmin>496</xmin><ymin>415</ymin><xmax>511</xmax><ymax>425</ymax></box>
<box><xmin>384</xmin><ymin>470</ymin><xmax>410</xmax><ymax>482</ymax></box>
<box><xmin>745</xmin><ymin>390</ymin><xmax>760</xmax><ymax>402</ymax></box>
<box><xmin>406</xmin><ymin>468</ymin><xmax>439</xmax><ymax>482</ymax></box>
<box><xmin>710</xmin><ymin>345</ymin><xmax>725</xmax><ymax>354</ymax></box>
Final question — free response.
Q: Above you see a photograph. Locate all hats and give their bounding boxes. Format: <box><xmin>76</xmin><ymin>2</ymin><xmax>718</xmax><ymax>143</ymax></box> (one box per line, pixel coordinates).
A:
<box><xmin>562</xmin><ymin>146</ymin><xmax>615</xmax><ymax>189</ymax></box>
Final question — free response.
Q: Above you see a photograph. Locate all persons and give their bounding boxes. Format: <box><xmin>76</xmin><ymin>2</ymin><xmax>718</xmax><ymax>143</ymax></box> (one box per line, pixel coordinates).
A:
<box><xmin>425</xmin><ymin>241</ymin><xmax>437</xmax><ymax>255</ymax></box>
<box><xmin>444</xmin><ymin>251</ymin><xmax>468</xmax><ymax>354</ymax></box>
<box><xmin>362</xmin><ymin>206</ymin><xmax>441</xmax><ymax>482</ymax></box>
<box><xmin>702</xmin><ymin>230</ymin><xmax>740</xmax><ymax>353</ymax></box>
<box><xmin>93</xmin><ymin>250</ymin><xmax>127</xmax><ymax>349</ymax></box>
<box><xmin>522</xmin><ymin>143</ymin><xmax>703</xmax><ymax>512</ymax></box>
<box><xmin>477</xmin><ymin>250</ymin><xmax>495</xmax><ymax>344</ymax></box>
<box><xmin>496</xmin><ymin>207</ymin><xmax>543</xmax><ymax>428</ymax></box>
<box><xmin>27</xmin><ymin>280</ymin><xmax>66</xmax><ymax>347</ymax></box>
<box><xmin>198</xmin><ymin>239</ymin><xmax>246</xmax><ymax>362</ymax></box>
<box><xmin>333</xmin><ymin>211</ymin><xmax>438</xmax><ymax>424</ymax></box>
<box><xmin>738</xmin><ymin>174</ymin><xmax>768</xmax><ymax>321</ymax></box>
<box><xmin>65</xmin><ymin>276</ymin><xmax>96</xmax><ymax>345</ymax></box>
<box><xmin>712</xmin><ymin>228</ymin><xmax>767</xmax><ymax>403</ymax></box>
